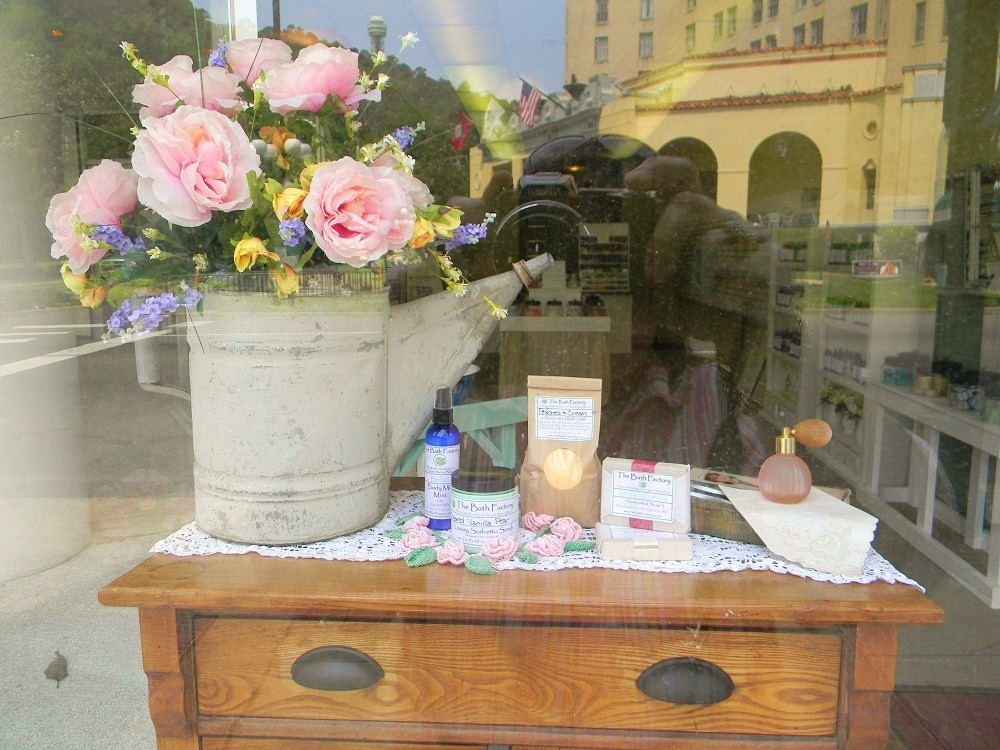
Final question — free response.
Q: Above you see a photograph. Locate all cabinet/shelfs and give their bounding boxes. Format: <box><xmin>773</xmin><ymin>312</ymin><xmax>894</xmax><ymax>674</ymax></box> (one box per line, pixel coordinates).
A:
<box><xmin>660</xmin><ymin>165</ymin><xmax>1000</xmax><ymax>614</ymax></box>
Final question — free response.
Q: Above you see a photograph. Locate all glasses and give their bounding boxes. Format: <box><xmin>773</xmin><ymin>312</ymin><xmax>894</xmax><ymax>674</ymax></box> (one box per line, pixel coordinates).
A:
<box><xmin>527</xmin><ymin>134</ymin><xmax>655</xmax><ymax>185</ymax></box>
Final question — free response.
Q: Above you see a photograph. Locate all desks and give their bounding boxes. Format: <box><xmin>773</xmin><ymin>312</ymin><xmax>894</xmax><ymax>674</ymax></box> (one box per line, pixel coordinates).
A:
<box><xmin>97</xmin><ymin>476</ymin><xmax>946</xmax><ymax>750</ymax></box>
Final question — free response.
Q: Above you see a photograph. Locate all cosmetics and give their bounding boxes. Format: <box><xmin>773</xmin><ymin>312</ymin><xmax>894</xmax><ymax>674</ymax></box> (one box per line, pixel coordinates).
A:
<box><xmin>450</xmin><ymin>465</ymin><xmax>520</xmax><ymax>555</ymax></box>
<box><xmin>422</xmin><ymin>383</ymin><xmax>462</xmax><ymax>530</ymax></box>
<box><xmin>760</xmin><ymin>418</ymin><xmax>831</xmax><ymax>504</ymax></box>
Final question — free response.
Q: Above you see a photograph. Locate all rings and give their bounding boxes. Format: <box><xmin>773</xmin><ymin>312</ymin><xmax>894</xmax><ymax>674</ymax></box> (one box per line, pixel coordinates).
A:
<box><xmin>723</xmin><ymin>219</ymin><xmax>760</xmax><ymax>254</ymax></box>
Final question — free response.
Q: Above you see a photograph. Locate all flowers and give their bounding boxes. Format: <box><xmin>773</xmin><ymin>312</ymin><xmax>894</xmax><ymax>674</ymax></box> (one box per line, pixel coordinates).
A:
<box><xmin>0</xmin><ymin>5</ymin><xmax>510</xmax><ymax>346</ymax></box>
<box><xmin>385</xmin><ymin>510</ymin><xmax>597</xmax><ymax>576</ymax></box>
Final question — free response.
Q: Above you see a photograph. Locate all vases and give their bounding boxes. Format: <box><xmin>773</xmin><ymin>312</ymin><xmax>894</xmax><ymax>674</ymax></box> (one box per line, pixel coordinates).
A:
<box><xmin>183</xmin><ymin>288</ymin><xmax>391</xmax><ymax>546</ymax></box>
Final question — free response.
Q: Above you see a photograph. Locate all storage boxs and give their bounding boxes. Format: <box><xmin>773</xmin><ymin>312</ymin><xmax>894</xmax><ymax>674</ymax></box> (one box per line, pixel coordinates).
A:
<box><xmin>689</xmin><ymin>467</ymin><xmax>851</xmax><ymax>554</ymax></box>
<box><xmin>600</xmin><ymin>456</ymin><xmax>693</xmax><ymax>534</ymax></box>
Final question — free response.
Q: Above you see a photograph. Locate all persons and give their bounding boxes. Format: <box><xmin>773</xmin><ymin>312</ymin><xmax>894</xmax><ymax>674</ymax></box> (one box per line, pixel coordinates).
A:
<box><xmin>442</xmin><ymin>133</ymin><xmax>957</xmax><ymax>539</ymax></box>
<box><xmin>698</xmin><ymin>472</ymin><xmax>759</xmax><ymax>490</ymax></box>
